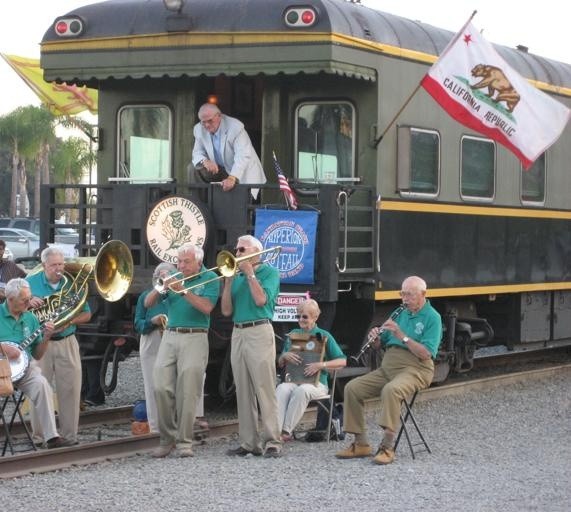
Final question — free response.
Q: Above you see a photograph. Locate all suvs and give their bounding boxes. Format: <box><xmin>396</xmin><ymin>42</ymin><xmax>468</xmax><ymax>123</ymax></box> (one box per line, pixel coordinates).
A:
<box><xmin>0</xmin><ymin>215</ymin><xmax>95</xmax><ymax>247</ymax></box>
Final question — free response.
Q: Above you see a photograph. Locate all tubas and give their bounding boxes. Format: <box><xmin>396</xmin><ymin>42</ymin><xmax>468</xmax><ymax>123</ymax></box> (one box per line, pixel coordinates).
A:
<box><xmin>24</xmin><ymin>240</ymin><xmax>133</xmax><ymax>330</ymax></box>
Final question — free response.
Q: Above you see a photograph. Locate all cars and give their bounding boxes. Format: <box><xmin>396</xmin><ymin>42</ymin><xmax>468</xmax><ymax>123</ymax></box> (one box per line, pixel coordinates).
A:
<box><xmin>72</xmin><ymin>221</ymin><xmax>96</xmax><ymax>240</ymax></box>
<box><xmin>1</xmin><ymin>228</ymin><xmax>80</xmax><ymax>266</ymax></box>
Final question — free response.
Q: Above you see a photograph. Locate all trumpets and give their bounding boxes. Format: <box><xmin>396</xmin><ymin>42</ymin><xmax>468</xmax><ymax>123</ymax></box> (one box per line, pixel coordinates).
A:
<box><xmin>151</xmin><ymin>271</ymin><xmax>184</xmax><ymax>292</ymax></box>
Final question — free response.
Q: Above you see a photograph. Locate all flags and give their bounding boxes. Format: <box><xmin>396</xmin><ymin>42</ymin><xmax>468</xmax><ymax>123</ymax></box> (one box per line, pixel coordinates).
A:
<box><xmin>3</xmin><ymin>54</ymin><xmax>99</xmax><ymax>116</ymax></box>
<box><xmin>272</xmin><ymin>155</ymin><xmax>298</xmax><ymax>210</ymax></box>
<box><xmin>419</xmin><ymin>19</ymin><xmax>571</xmax><ymax>171</ymax></box>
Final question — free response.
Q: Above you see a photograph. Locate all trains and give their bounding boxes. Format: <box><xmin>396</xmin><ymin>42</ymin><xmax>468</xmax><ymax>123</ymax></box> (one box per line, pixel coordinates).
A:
<box><xmin>30</xmin><ymin>0</ymin><xmax>571</xmax><ymax>410</ymax></box>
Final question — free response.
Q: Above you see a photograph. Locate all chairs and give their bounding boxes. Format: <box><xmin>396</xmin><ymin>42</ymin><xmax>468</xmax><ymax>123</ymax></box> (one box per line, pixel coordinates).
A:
<box><xmin>291</xmin><ymin>366</ymin><xmax>341</xmax><ymax>444</ymax></box>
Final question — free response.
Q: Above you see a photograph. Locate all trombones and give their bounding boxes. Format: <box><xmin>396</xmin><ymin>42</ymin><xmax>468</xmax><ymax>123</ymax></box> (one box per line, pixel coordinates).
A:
<box><xmin>166</xmin><ymin>246</ymin><xmax>281</xmax><ymax>294</ymax></box>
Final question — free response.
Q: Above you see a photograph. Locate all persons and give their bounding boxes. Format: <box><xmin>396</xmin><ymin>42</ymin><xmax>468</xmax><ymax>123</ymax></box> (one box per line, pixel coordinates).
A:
<box><xmin>0</xmin><ymin>240</ymin><xmax>28</xmax><ymax>304</ymax></box>
<box><xmin>133</xmin><ymin>262</ymin><xmax>208</xmax><ymax>435</ymax></box>
<box><xmin>151</xmin><ymin>243</ymin><xmax>220</xmax><ymax>458</ymax></box>
<box><xmin>276</xmin><ymin>299</ymin><xmax>347</xmax><ymax>441</ymax></box>
<box><xmin>221</xmin><ymin>235</ymin><xmax>283</xmax><ymax>458</ymax></box>
<box><xmin>333</xmin><ymin>275</ymin><xmax>442</xmax><ymax>465</ymax></box>
<box><xmin>0</xmin><ymin>278</ymin><xmax>78</xmax><ymax>449</ymax></box>
<box><xmin>25</xmin><ymin>247</ymin><xmax>92</xmax><ymax>447</ymax></box>
<box><xmin>186</xmin><ymin>103</ymin><xmax>267</xmax><ymax>204</ymax></box>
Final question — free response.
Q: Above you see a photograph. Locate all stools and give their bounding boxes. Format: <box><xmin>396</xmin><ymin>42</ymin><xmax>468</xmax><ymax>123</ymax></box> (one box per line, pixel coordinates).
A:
<box><xmin>392</xmin><ymin>388</ymin><xmax>431</xmax><ymax>459</ymax></box>
<box><xmin>0</xmin><ymin>383</ymin><xmax>38</xmax><ymax>459</ymax></box>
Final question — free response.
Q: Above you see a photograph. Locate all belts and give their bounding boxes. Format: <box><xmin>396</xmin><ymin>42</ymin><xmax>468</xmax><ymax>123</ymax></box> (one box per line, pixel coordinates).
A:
<box><xmin>166</xmin><ymin>327</ymin><xmax>209</xmax><ymax>334</ymax></box>
<box><xmin>49</xmin><ymin>333</ymin><xmax>74</xmax><ymax>341</ymax></box>
<box><xmin>234</xmin><ymin>319</ymin><xmax>272</xmax><ymax>329</ymax></box>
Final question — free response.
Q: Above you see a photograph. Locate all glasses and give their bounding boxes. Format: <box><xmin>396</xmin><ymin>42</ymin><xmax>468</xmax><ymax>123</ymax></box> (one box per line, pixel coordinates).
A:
<box><xmin>295</xmin><ymin>314</ymin><xmax>311</xmax><ymax>319</ymax></box>
<box><xmin>233</xmin><ymin>246</ymin><xmax>254</xmax><ymax>253</ymax></box>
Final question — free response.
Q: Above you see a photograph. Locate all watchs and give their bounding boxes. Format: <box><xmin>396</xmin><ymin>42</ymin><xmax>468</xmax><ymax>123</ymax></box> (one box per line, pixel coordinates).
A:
<box><xmin>402</xmin><ymin>336</ymin><xmax>409</xmax><ymax>344</ymax></box>
<box><xmin>180</xmin><ymin>290</ymin><xmax>189</xmax><ymax>296</ymax></box>
<box><xmin>247</xmin><ymin>275</ymin><xmax>255</xmax><ymax>280</ymax></box>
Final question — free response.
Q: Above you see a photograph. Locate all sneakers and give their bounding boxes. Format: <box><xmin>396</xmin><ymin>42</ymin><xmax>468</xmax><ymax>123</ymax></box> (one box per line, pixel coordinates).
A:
<box><xmin>225</xmin><ymin>445</ymin><xmax>262</xmax><ymax>457</ymax></box>
<box><xmin>336</xmin><ymin>444</ymin><xmax>371</xmax><ymax>458</ymax></box>
<box><xmin>151</xmin><ymin>443</ymin><xmax>175</xmax><ymax>458</ymax></box>
<box><xmin>262</xmin><ymin>445</ymin><xmax>283</xmax><ymax>459</ymax></box>
<box><xmin>373</xmin><ymin>447</ymin><xmax>397</xmax><ymax>464</ymax></box>
<box><xmin>32</xmin><ymin>438</ymin><xmax>79</xmax><ymax>450</ymax></box>
<box><xmin>174</xmin><ymin>444</ymin><xmax>195</xmax><ymax>458</ymax></box>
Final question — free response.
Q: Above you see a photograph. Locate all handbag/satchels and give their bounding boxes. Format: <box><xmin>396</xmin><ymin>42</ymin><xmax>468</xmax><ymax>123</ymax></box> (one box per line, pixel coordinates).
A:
<box><xmin>304</xmin><ymin>398</ymin><xmax>347</xmax><ymax>442</ymax></box>
<box><xmin>0</xmin><ymin>349</ymin><xmax>14</xmax><ymax>398</ymax></box>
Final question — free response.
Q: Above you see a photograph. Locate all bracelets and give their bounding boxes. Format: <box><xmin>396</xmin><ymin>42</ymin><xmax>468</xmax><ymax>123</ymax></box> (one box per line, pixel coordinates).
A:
<box><xmin>69</xmin><ymin>321</ymin><xmax>73</xmax><ymax>326</ymax></box>
<box><xmin>323</xmin><ymin>362</ymin><xmax>327</xmax><ymax>369</ymax></box>
<box><xmin>228</xmin><ymin>176</ymin><xmax>236</xmax><ymax>181</ymax></box>
